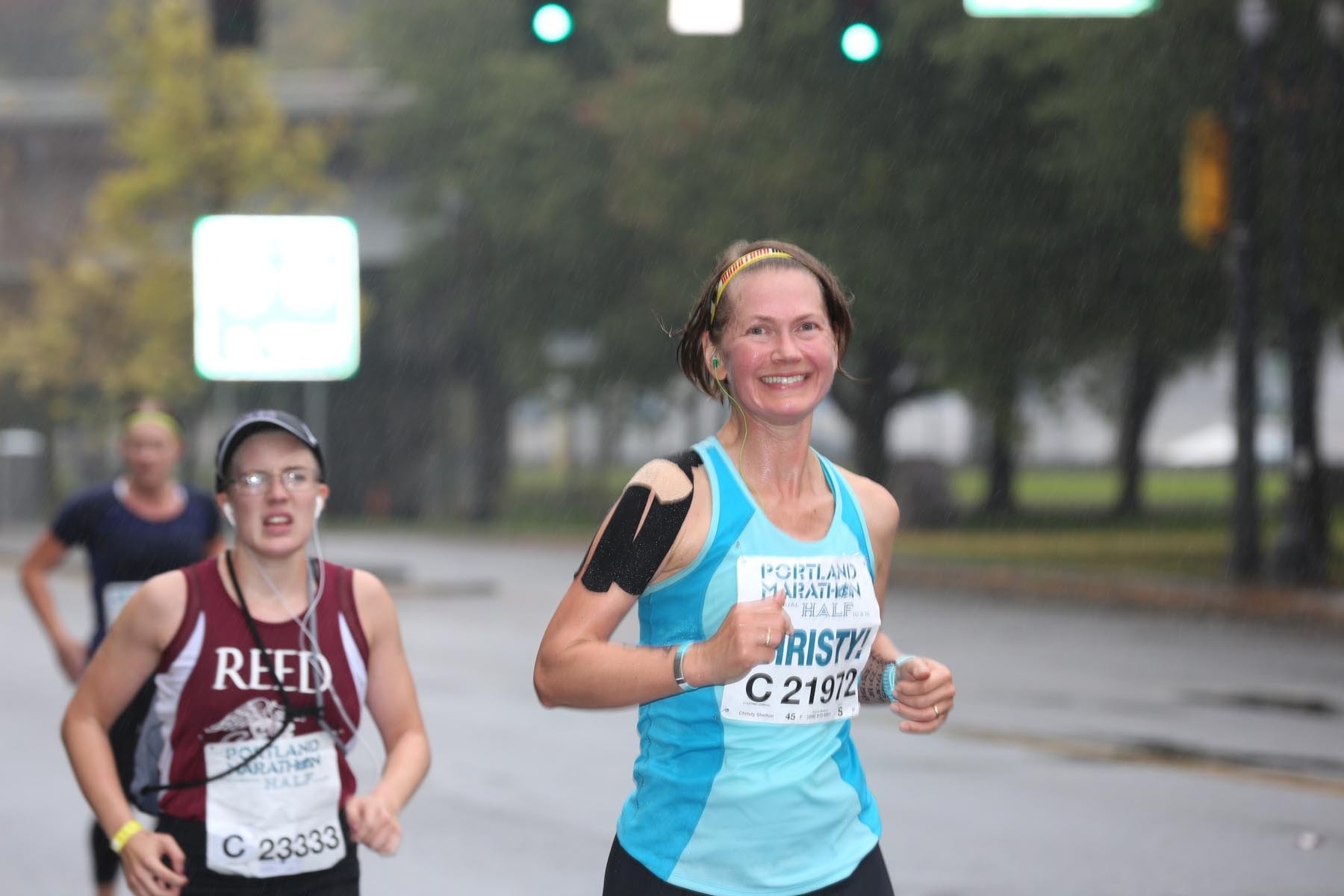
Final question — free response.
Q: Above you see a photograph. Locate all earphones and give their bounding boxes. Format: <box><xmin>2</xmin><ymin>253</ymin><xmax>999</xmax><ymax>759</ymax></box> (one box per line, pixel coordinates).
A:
<box><xmin>313</xmin><ymin>494</ymin><xmax>325</xmax><ymax>520</ymax></box>
<box><xmin>223</xmin><ymin>502</ymin><xmax>238</xmax><ymax>527</ymax></box>
<box><xmin>712</xmin><ymin>356</ymin><xmax>720</xmax><ymax>370</ymax></box>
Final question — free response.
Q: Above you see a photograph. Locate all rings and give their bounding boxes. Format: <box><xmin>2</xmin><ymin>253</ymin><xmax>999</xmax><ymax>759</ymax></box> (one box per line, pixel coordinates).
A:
<box><xmin>933</xmin><ymin>704</ymin><xmax>939</xmax><ymax>716</ymax></box>
<box><xmin>765</xmin><ymin>628</ymin><xmax>772</xmax><ymax>644</ymax></box>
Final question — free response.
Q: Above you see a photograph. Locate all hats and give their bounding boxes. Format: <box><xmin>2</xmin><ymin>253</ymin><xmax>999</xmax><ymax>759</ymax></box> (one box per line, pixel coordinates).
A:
<box><xmin>213</xmin><ymin>409</ymin><xmax>321</xmax><ymax>479</ymax></box>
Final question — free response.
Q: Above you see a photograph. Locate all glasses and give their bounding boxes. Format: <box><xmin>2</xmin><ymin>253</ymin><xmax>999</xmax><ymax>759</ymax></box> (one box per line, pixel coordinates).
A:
<box><xmin>226</xmin><ymin>469</ymin><xmax>319</xmax><ymax>495</ymax></box>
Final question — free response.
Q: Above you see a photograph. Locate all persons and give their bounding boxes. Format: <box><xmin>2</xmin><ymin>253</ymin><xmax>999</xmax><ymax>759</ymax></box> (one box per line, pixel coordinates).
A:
<box><xmin>60</xmin><ymin>410</ymin><xmax>434</xmax><ymax>896</ymax></box>
<box><xmin>534</xmin><ymin>236</ymin><xmax>954</xmax><ymax>896</ymax></box>
<box><xmin>18</xmin><ymin>402</ymin><xmax>223</xmax><ymax>896</ymax></box>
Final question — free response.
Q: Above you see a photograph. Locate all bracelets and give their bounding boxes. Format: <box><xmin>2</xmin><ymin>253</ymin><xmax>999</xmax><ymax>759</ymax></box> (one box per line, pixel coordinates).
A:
<box><xmin>108</xmin><ymin>820</ymin><xmax>143</xmax><ymax>855</ymax></box>
<box><xmin>672</xmin><ymin>639</ymin><xmax>706</xmax><ymax>691</ymax></box>
<box><xmin>881</xmin><ymin>654</ymin><xmax>915</xmax><ymax>704</ymax></box>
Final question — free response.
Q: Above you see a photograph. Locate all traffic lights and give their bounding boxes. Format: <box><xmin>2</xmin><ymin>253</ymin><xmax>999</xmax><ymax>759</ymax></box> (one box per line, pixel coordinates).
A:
<box><xmin>527</xmin><ymin>0</ymin><xmax>574</xmax><ymax>48</ymax></box>
<box><xmin>841</xmin><ymin>1</ymin><xmax>885</xmax><ymax>66</ymax></box>
